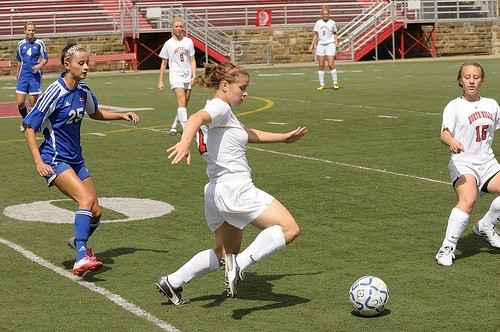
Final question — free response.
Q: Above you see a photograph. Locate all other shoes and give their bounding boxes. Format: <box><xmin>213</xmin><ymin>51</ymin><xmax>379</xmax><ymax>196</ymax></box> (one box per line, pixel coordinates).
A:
<box><xmin>20</xmin><ymin>121</ymin><xmax>26</xmax><ymax>132</ymax></box>
<box><xmin>317</xmin><ymin>85</ymin><xmax>325</xmax><ymax>90</ymax></box>
<box><xmin>334</xmin><ymin>83</ymin><xmax>339</xmax><ymax>89</ymax></box>
<box><xmin>170</xmin><ymin>126</ymin><xmax>177</xmax><ymax>133</ymax></box>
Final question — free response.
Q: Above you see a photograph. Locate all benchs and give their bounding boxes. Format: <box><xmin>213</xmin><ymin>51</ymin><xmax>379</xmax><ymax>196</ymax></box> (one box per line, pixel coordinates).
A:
<box><xmin>0</xmin><ymin>53</ymin><xmax>137</xmax><ymax>75</ymax></box>
<box><xmin>0</xmin><ymin>0</ymin><xmax>490</xmax><ymax>35</ymax></box>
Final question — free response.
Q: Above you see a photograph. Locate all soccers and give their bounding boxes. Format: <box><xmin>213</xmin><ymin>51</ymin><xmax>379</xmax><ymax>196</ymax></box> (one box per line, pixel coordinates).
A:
<box><xmin>348</xmin><ymin>276</ymin><xmax>389</xmax><ymax>317</ymax></box>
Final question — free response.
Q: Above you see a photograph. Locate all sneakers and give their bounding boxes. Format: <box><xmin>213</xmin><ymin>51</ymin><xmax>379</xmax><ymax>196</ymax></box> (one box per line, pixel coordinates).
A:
<box><xmin>155</xmin><ymin>275</ymin><xmax>190</xmax><ymax>306</ymax></box>
<box><xmin>220</xmin><ymin>253</ymin><xmax>244</xmax><ymax>298</ymax></box>
<box><xmin>436</xmin><ymin>246</ymin><xmax>455</xmax><ymax>266</ymax></box>
<box><xmin>68</xmin><ymin>237</ymin><xmax>97</xmax><ymax>260</ymax></box>
<box><xmin>73</xmin><ymin>256</ymin><xmax>103</xmax><ymax>276</ymax></box>
<box><xmin>472</xmin><ymin>219</ymin><xmax>500</xmax><ymax>248</ymax></box>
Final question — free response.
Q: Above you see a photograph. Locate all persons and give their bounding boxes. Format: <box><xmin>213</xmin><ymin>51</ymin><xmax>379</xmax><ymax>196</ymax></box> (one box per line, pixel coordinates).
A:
<box><xmin>435</xmin><ymin>62</ymin><xmax>500</xmax><ymax>267</ymax></box>
<box><xmin>309</xmin><ymin>6</ymin><xmax>340</xmax><ymax>90</ymax></box>
<box><xmin>155</xmin><ymin>62</ymin><xmax>307</xmax><ymax>305</ymax></box>
<box><xmin>17</xmin><ymin>23</ymin><xmax>48</xmax><ymax>133</ymax></box>
<box><xmin>22</xmin><ymin>44</ymin><xmax>139</xmax><ymax>276</ymax></box>
<box><xmin>158</xmin><ymin>20</ymin><xmax>196</xmax><ymax>134</ymax></box>
